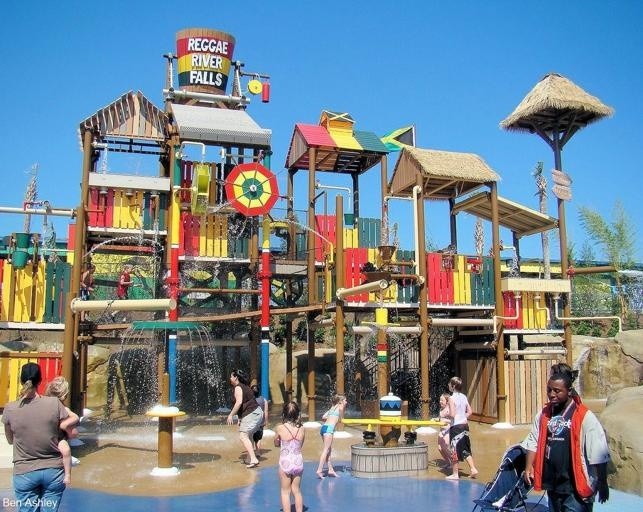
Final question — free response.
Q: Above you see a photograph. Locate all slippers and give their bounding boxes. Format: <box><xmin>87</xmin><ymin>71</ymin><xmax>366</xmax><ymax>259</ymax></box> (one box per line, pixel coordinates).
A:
<box><xmin>240</xmin><ymin>449</ymin><xmax>261</xmax><ymax>469</ymax></box>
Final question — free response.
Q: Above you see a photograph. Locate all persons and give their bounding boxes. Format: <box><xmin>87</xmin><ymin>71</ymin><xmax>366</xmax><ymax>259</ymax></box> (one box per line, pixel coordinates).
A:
<box><xmin>315</xmin><ymin>393</ymin><xmax>348</xmax><ymax>480</ymax></box>
<box><xmin>227</xmin><ymin>368</ymin><xmax>269</xmax><ymax>469</ymax></box>
<box><xmin>519</xmin><ymin>363</ymin><xmax>617</xmax><ymax>512</ymax></box>
<box><xmin>109</xmin><ymin>264</ymin><xmax>134</xmax><ymax>324</ymax></box>
<box><xmin>273</xmin><ymin>402</ymin><xmax>306</xmax><ymax>511</ymax></box>
<box><xmin>80</xmin><ymin>263</ymin><xmax>96</xmax><ymax>323</ymax></box>
<box><xmin>437</xmin><ymin>376</ymin><xmax>478</xmax><ymax>481</ymax></box>
<box><xmin>1</xmin><ymin>362</ymin><xmax>79</xmax><ymax>512</ymax></box>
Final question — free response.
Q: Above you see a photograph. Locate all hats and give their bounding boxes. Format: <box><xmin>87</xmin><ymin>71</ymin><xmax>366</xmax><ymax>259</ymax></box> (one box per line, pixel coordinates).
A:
<box><xmin>20</xmin><ymin>363</ymin><xmax>41</xmax><ymax>384</ymax></box>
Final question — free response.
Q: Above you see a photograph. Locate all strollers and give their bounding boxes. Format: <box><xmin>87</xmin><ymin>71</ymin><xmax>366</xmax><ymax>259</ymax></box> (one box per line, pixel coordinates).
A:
<box><xmin>471</xmin><ymin>441</ymin><xmax>546</xmax><ymax>512</ymax></box>
